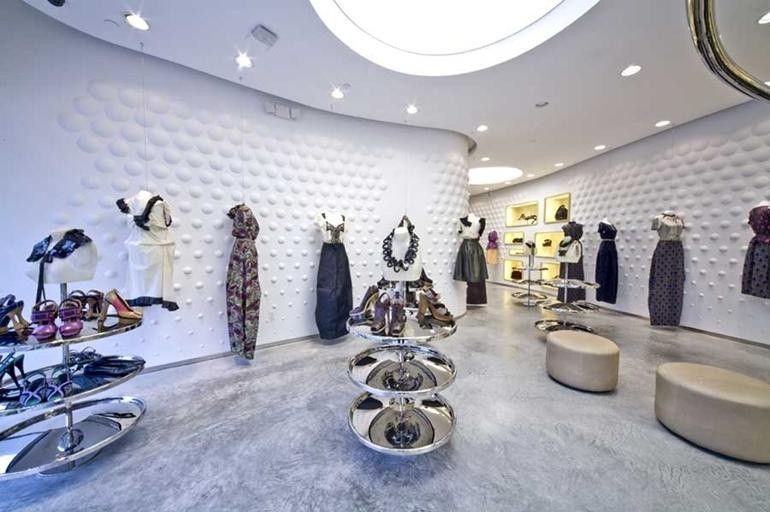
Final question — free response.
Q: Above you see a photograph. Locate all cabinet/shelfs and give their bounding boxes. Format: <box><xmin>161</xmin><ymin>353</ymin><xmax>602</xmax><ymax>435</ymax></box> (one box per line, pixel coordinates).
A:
<box><xmin>345</xmin><ymin>311</ymin><xmax>458</xmax><ymax>457</ymax></box>
<box><xmin>0</xmin><ymin>285</ymin><xmax>153</xmax><ymax>484</ymax></box>
<box><xmin>533</xmin><ymin>280</ymin><xmax>602</xmax><ymax>337</ymax></box>
<box><xmin>509</xmin><ymin>263</ymin><xmax>550</xmax><ymax>310</ymax></box>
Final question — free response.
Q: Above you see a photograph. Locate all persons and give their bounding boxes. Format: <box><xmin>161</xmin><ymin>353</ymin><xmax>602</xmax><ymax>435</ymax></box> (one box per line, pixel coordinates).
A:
<box><xmin>114</xmin><ymin>190</ymin><xmax>179</xmax><ymax>311</ymax></box>
<box><xmin>648</xmin><ymin>210</ymin><xmax>686</xmax><ymax>325</ymax></box>
<box><xmin>741</xmin><ymin>200</ymin><xmax>770</xmax><ymax>298</ymax></box>
<box><xmin>486</xmin><ymin>229</ymin><xmax>498</xmax><ymax>264</ymax></box>
<box><xmin>455</xmin><ymin>213</ymin><xmax>489</xmax><ymax>282</ymax></box>
<box><xmin>595</xmin><ymin>216</ymin><xmax>617</xmax><ymax>303</ymax></box>
<box><xmin>316</xmin><ymin>207</ymin><xmax>354</xmax><ymax>338</ymax></box>
<box><xmin>228</xmin><ymin>204</ymin><xmax>261</xmax><ymax>359</ymax></box>
<box><xmin>22</xmin><ymin>227</ymin><xmax>98</xmax><ymax>285</ymax></box>
<box><xmin>558</xmin><ymin>218</ymin><xmax>585</xmax><ymax>303</ymax></box>
<box><xmin>379</xmin><ymin>226</ymin><xmax>425</xmax><ymax>282</ymax></box>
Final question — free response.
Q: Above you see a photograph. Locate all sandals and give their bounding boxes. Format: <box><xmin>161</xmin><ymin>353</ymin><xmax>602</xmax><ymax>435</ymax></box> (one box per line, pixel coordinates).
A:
<box><xmin>1</xmin><ymin>346</ymin><xmax>146</xmax><ymax>407</ymax></box>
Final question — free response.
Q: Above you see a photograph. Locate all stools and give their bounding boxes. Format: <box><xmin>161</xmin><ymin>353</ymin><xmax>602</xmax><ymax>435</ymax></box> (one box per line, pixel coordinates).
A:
<box><xmin>542</xmin><ymin>329</ymin><xmax>621</xmax><ymax>392</ymax></box>
<box><xmin>654</xmin><ymin>360</ymin><xmax>768</xmax><ymax>470</ymax></box>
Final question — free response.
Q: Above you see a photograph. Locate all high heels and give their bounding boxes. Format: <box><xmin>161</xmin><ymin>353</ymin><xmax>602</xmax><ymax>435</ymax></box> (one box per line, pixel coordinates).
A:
<box><xmin>0</xmin><ymin>290</ymin><xmax>142</xmax><ymax>342</ymax></box>
<box><xmin>348</xmin><ymin>268</ymin><xmax>455</xmax><ymax>336</ymax></box>
